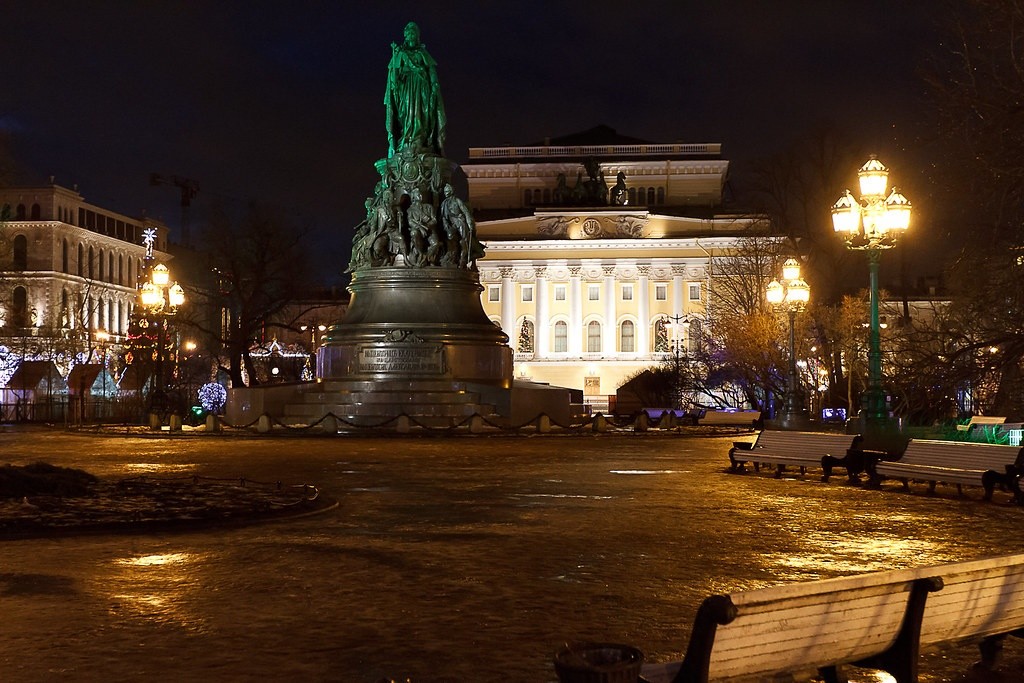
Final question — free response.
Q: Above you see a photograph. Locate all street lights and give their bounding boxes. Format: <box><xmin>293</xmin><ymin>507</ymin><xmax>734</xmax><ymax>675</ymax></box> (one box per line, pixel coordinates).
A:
<box><xmin>969</xmin><ymin>347</ymin><xmax>998</xmax><ymax>412</ymax></box>
<box><xmin>831</xmin><ymin>156</ymin><xmax>912</xmax><ymax>437</ymax></box>
<box><xmin>766</xmin><ymin>257</ymin><xmax>812</xmax><ymax>431</ymax></box>
<box><xmin>664</xmin><ymin>314</ymin><xmax>692</xmax><ymax>408</ymax></box>
<box><xmin>301</xmin><ymin>319</ymin><xmax>327</xmax><ymax>376</ymax></box>
<box><xmin>97</xmin><ymin>332</ymin><xmax>111</xmax><ymax>420</ymax></box>
<box><xmin>140</xmin><ymin>265</ymin><xmax>185</xmax><ymax>427</ymax></box>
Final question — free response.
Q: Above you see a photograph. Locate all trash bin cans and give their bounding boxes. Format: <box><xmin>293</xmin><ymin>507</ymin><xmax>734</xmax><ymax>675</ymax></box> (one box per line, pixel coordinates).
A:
<box><xmin>553</xmin><ymin>642</ymin><xmax>643</xmax><ymax>683</ymax></box>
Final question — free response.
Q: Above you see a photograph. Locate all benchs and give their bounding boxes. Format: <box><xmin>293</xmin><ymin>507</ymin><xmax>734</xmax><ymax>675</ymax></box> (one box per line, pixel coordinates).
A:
<box><xmin>696</xmin><ymin>409</ymin><xmax>763</xmax><ymax>428</ymax></box>
<box><xmin>956</xmin><ymin>415</ymin><xmax>1024</xmax><ymax>440</ymax></box>
<box><xmin>867</xmin><ymin>437</ymin><xmax>1024</xmax><ymax>506</ymax></box>
<box><xmin>728</xmin><ymin>430</ymin><xmax>864</xmax><ymax>486</ymax></box>
<box><xmin>627</xmin><ymin>551</ymin><xmax>1024</xmax><ymax>683</ymax></box>
